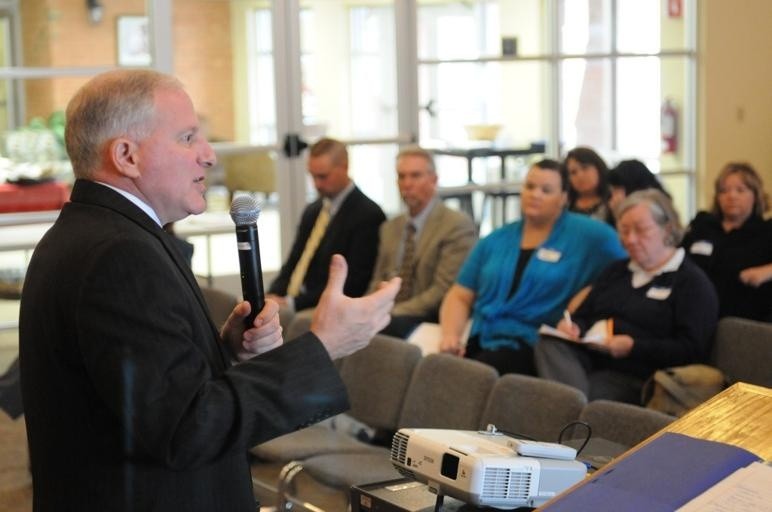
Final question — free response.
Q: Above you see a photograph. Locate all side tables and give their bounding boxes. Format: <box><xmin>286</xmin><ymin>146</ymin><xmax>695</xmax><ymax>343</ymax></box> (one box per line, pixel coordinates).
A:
<box><xmin>426</xmin><ymin>141</ymin><xmax>547</xmax><ymax>235</ymax></box>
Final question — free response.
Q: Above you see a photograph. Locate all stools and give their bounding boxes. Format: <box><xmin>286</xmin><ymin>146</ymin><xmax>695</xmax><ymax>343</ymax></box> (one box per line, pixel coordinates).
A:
<box><xmin>435</xmin><ymin>182</ymin><xmax>478</xmax><ymax>221</ymax></box>
<box><xmin>478</xmin><ymin>183</ymin><xmax>525</xmax><ymax>232</ymax></box>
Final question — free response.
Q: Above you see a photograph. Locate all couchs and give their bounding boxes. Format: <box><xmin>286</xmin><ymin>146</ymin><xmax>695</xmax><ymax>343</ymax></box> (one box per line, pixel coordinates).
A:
<box><xmin>223</xmin><ymin>148</ymin><xmax>277</xmax><ymax>204</ymax></box>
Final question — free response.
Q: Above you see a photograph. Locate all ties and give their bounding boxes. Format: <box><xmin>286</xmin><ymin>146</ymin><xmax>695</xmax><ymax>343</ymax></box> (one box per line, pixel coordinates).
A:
<box><xmin>394</xmin><ymin>224</ymin><xmax>417</xmax><ymax>304</ymax></box>
<box><xmin>286</xmin><ymin>203</ymin><xmax>333</xmax><ymax>302</ymax></box>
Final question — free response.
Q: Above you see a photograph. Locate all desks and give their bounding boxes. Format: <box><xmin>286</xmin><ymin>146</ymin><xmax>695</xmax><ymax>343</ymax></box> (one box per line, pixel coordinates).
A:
<box><xmin>528</xmin><ymin>382</ymin><xmax>772</xmax><ymax>512</ymax></box>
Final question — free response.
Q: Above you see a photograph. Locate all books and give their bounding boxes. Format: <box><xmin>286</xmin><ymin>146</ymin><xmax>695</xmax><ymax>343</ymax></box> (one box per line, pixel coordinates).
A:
<box><xmin>539</xmin><ymin>317</ymin><xmax>615</xmax><ymax>345</ymax></box>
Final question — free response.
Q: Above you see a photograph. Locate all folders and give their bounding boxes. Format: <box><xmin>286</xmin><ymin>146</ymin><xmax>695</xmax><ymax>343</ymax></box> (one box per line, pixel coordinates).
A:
<box><xmin>541</xmin><ymin>431</ymin><xmax>771</xmax><ymax>512</ymax></box>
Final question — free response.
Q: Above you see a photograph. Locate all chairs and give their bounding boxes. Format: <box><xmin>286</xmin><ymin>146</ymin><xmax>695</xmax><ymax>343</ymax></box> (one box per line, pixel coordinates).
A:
<box><xmin>199</xmin><ymin>287</ymin><xmax>236</xmax><ymax>329</ymax></box>
<box><xmin>483</xmin><ymin>372</ymin><xmax>584</xmax><ymax>446</ymax></box>
<box><xmin>713</xmin><ymin>315</ymin><xmax>771</xmax><ymax>386</ymax></box>
<box><xmin>278</xmin><ymin>351</ymin><xmax>498</xmax><ymax>512</ymax></box>
<box><xmin>572</xmin><ymin>400</ymin><xmax>675</xmax><ymax>468</ymax></box>
<box><xmin>249</xmin><ymin>330</ymin><xmax>421</xmax><ymax>512</ymax></box>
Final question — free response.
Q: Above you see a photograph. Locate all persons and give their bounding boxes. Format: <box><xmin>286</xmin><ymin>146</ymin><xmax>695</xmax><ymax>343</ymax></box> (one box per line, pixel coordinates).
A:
<box><xmin>363</xmin><ymin>142</ymin><xmax>479</xmax><ymax>341</ymax></box>
<box><xmin>439</xmin><ymin>158</ymin><xmax>631</xmax><ymax>378</ymax></box>
<box><xmin>264</xmin><ymin>139</ymin><xmax>387</xmax><ymax>315</ymax></box>
<box><xmin>604</xmin><ymin>159</ymin><xmax>669</xmax><ymax>224</ymax></box>
<box><xmin>532</xmin><ymin>188</ymin><xmax>721</xmax><ymax>409</ymax></box>
<box><xmin>564</xmin><ymin>148</ymin><xmax>618</xmax><ymax>230</ymax></box>
<box><xmin>19</xmin><ymin>69</ymin><xmax>402</xmax><ymax>512</ymax></box>
<box><xmin>676</xmin><ymin>161</ymin><xmax>772</xmax><ymax>324</ymax></box>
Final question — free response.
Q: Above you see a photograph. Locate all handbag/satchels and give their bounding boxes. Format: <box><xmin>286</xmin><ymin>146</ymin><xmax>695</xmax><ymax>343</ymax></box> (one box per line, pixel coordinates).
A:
<box><xmin>645</xmin><ymin>364</ymin><xmax>725</xmax><ymax>421</ymax></box>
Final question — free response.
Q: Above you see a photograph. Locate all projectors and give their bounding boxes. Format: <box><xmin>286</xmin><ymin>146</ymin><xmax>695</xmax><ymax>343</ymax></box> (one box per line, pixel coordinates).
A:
<box><xmin>387</xmin><ymin>421</ymin><xmax>587</xmax><ymax>510</ymax></box>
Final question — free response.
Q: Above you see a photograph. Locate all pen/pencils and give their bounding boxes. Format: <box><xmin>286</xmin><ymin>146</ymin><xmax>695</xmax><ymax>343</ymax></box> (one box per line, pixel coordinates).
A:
<box><xmin>563</xmin><ymin>309</ymin><xmax>572</xmax><ymax>327</ymax></box>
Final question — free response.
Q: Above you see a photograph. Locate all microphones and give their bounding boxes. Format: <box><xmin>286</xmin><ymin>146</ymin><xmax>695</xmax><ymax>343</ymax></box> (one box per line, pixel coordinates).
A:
<box><xmin>228</xmin><ymin>195</ymin><xmax>269</xmax><ymax>336</ymax></box>
